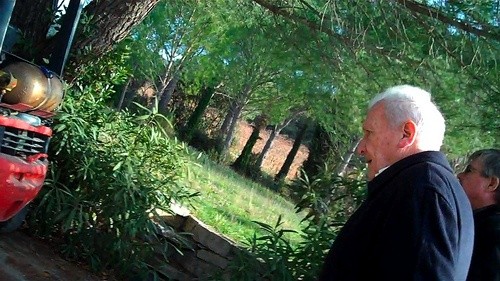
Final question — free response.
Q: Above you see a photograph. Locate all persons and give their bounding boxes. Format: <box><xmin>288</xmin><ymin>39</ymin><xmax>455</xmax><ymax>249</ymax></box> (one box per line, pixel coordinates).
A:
<box><xmin>318</xmin><ymin>84</ymin><xmax>474</xmax><ymax>281</ymax></box>
<box><xmin>457</xmin><ymin>148</ymin><xmax>500</xmax><ymax>281</ymax></box>
